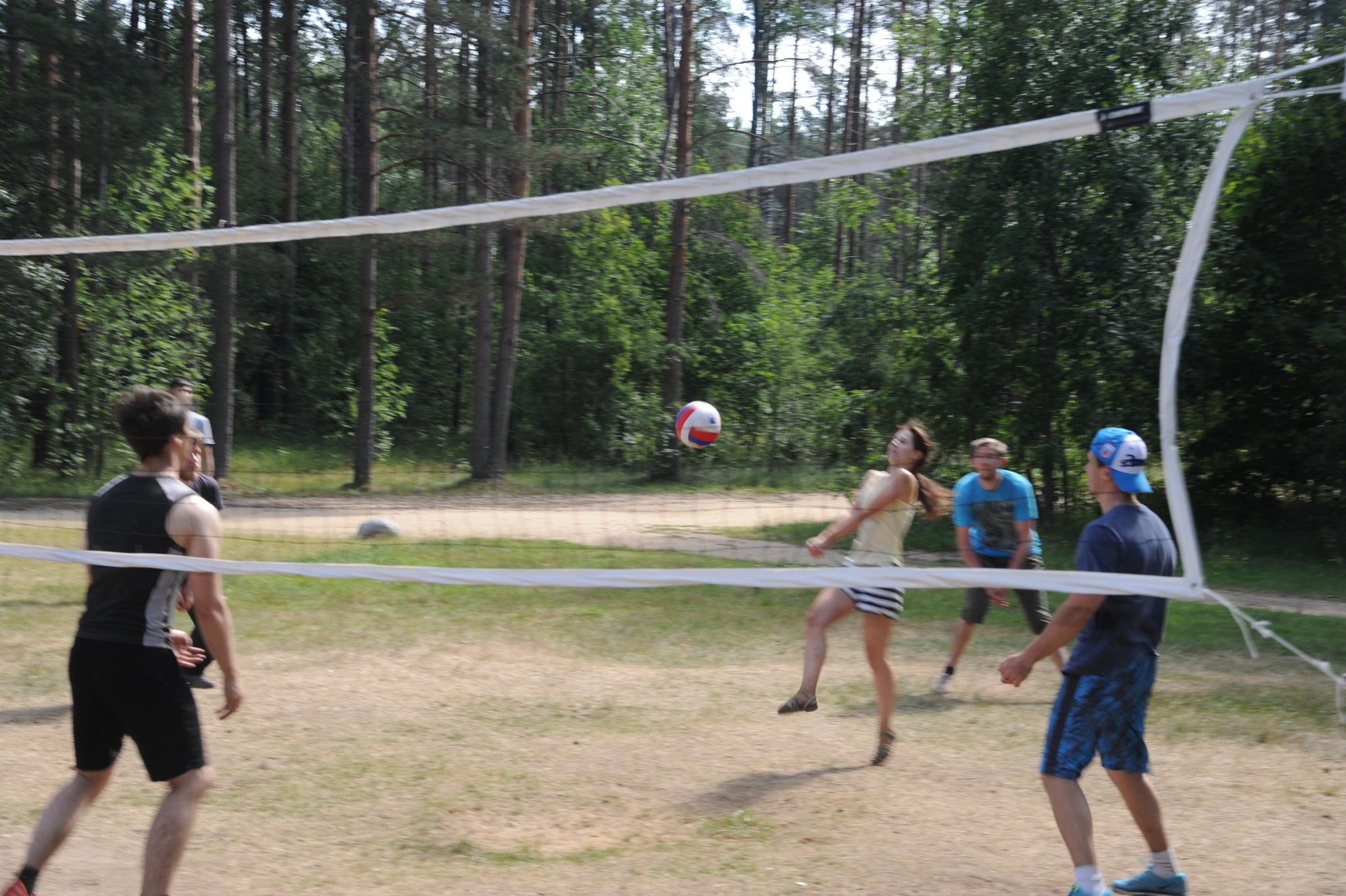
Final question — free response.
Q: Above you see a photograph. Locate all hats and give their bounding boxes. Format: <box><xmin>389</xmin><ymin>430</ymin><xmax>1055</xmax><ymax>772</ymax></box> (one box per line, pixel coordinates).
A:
<box><xmin>1089</xmin><ymin>427</ymin><xmax>1154</xmax><ymax>493</ymax></box>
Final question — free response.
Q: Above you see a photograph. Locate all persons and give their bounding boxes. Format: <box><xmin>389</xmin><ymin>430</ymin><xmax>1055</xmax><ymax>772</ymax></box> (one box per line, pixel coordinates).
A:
<box><xmin>921</xmin><ymin>437</ymin><xmax>1071</xmax><ymax>694</ymax></box>
<box><xmin>1</xmin><ymin>388</ymin><xmax>245</xmax><ymax>895</ymax></box>
<box><xmin>161</xmin><ymin>374</ymin><xmax>226</xmax><ymax>690</ymax></box>
<box><xmin>997</xmin><ymin>427</ymin><xmax>1188</xmax><ymax>896</ymax></box>
<box><xmin>777</xmin><ymin>418</ymin><xmax>961</xmax><ymax>767</ymax></box>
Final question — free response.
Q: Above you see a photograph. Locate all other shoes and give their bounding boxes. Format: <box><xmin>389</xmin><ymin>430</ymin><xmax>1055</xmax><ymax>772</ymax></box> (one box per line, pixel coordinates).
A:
<box><xmin>3</xmin><ymin>879</ymin><xmax>37</xmax><ymax>896</ymax></box>
<box><xmin>181</xmin><ymin>672</ymin><xmax>216</xmax><ymax>687</ymax></box>
<box><xmin>935</xmin><ymin>673</ymin><xmax>955</xmax><ymax>694</ymax></box>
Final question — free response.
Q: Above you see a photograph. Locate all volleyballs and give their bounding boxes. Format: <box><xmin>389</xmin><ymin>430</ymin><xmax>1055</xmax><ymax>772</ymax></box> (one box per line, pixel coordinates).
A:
<box><xmin>674</xmin><ymin>399</ymin><xmax>721</xmax><ymax>449</ymax></box>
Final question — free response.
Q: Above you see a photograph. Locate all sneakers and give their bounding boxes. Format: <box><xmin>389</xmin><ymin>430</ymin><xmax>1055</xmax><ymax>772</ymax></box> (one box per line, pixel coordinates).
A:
<box><xmin>1110</xmin><ymin>864</ymin><xmax>1188</xmax><ymax>896</ymax></box>
<box><xmin>1067</xmin><ymin>883</ymin><xmax>1113</xmax><ymax>896</ymax></box>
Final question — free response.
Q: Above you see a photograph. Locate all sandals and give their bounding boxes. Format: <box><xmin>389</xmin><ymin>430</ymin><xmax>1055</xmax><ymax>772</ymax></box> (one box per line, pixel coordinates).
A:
<box><xmin>874</xmin><ymin>729</ymin><xmax>897</xmax><ymax>763</ymax></box>
<box><xmin>778</xmin><ymin>690</ymin><xmax>818</xmax><ymax>713</ymax></box>
